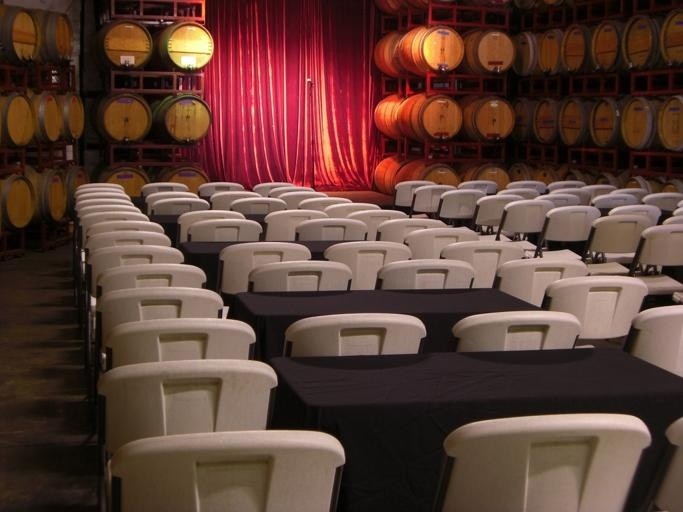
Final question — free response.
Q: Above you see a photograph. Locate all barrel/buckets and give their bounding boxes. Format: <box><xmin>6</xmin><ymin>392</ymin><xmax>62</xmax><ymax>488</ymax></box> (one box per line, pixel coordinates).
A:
<box><xmin>512</xmin><ymin>9</ymin><xmax>682</xmax><ymax>77</ymax></box>
<box><xmin>98</xmin><ymin>91</ymin><xmax>212</xmax><ymax>144</ymax></box>
<box><xmin>374</xmin><ymin>93</ymin><xmax>516</xmax><ymax>144</ymax></box>
<box><xmin>98</xmin><ymin>163</ymin><xmax>213</xmax><ymax>199</ymax></box>
<box><xmin>375</xmin><ymin>25</ymin><xmax>516</xmax><ymax>76</ymax></box>
<box><xmin>97</xmin><ymin>19</ymin><xmax>214</xmax><ymax>74</ymax></box>
<box><xmin>373</xmin><ymin>157</ymin><xmax>682</xmax><ymax>199</ymax></box>
<box><xmin>2</xmin><ymin>158</ymin><xmax>91</xmax><ymax>230</ymax></box>
<box><xmin>1</xmin><ymin>89</ymin><xmax>86</xmax><ymax>149</ymax></box>
<box><xmin>513</xmin><ymin>91</ymin><xmax>682</xmax><ymax>149</ymax></box>
<box><xmin>3</xmin><ymin>5</ymin><xmax>74</xmax><ymax>68</ymax></box>
